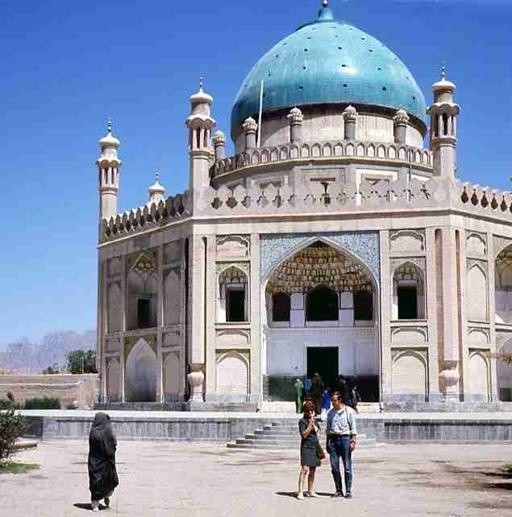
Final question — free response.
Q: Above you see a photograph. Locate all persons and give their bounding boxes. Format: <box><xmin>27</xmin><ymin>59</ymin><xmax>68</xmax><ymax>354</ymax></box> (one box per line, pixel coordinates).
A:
<box><xmin>296</xmin><ymin>402</ymin><xmax>324</xmax><ymax>501</ymax></box>
<box><xmin>87</xmin><ymin>411</ymin><xmax>120</xmax><ymax>512</ymax></box>
<box><xmin>294</xmin><ymin>372</ymin><xmax>361</xmax><ymax>415</ymax></box>
<box><xmin>323</xmin><ymin>391</ymin><xmax>359</xmax><ymax>500</ymax></box>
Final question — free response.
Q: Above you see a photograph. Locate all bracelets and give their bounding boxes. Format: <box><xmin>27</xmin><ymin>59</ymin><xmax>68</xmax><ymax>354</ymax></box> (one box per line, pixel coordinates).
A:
<box><xmin>350</xmin><ymin>438</ymin><xmax>356</xmax><ymax>443</ymax></box>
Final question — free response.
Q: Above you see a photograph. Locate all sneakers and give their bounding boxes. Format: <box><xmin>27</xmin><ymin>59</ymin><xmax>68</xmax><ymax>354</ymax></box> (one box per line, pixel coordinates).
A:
<box><xmin>297</xmin><ymin>491</ymin><xmax>321</xmax><ymax>500</ymax></box>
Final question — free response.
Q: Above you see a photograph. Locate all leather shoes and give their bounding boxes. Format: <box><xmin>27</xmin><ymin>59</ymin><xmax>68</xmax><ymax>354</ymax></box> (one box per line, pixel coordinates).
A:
<box><xmin>332</xmin><ymin>491</ymin><xmax>352</xmax><ymax>499</ymax></box>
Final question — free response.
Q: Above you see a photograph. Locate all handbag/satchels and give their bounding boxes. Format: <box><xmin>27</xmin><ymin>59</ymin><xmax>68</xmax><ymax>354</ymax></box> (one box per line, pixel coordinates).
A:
<box><xmin>316</xmin><ymin>445</ymin><xmax>325</xmax><ymax>459</ymax></box>
